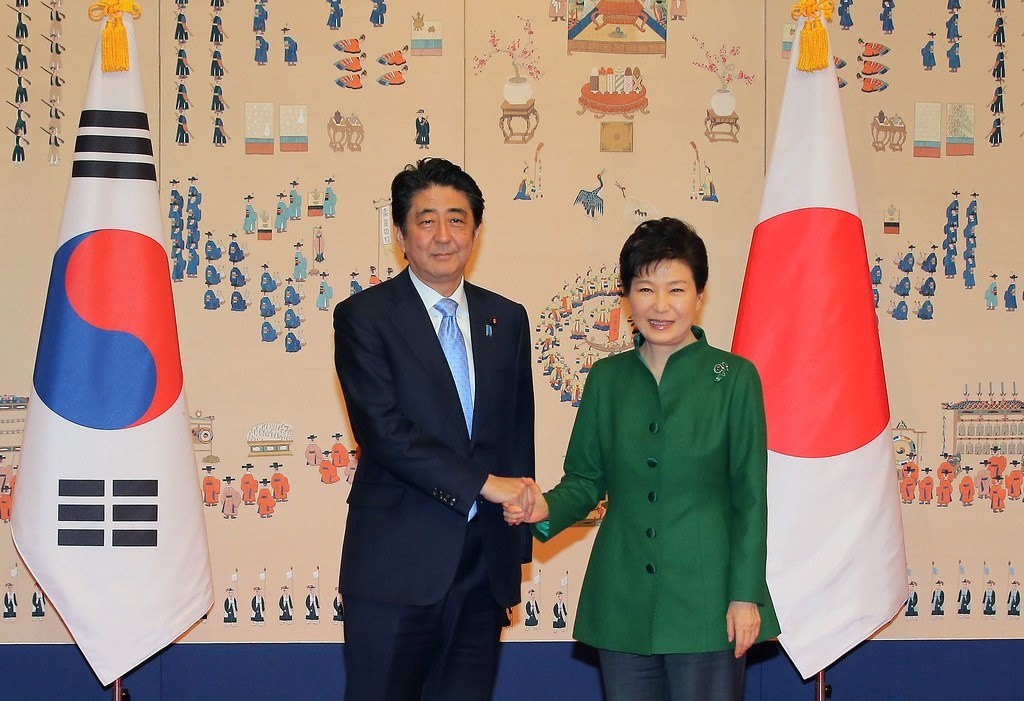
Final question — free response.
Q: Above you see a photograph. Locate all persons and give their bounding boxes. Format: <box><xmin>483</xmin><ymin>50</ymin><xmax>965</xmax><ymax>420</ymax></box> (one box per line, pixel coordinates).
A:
<box><xmin>501</xmin><ymin>217</ymin><xmax>781</xmax><ymax>701</ymax></box>
<box><xmin>333</xmin><ymin>156</ymin><xmax>536</xmax><ymax>701</ymax></box>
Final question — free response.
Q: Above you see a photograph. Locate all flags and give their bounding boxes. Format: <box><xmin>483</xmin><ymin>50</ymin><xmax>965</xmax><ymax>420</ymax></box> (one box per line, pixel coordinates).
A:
<box><xmin>732</xmin><ymin>0</ymin><xmax>910</xmax><ymax>681</ymax></box>
<box><xmin>10</xmin><ymin>0</ymin><xmax>215</xmax><ymax>686</ymax></box>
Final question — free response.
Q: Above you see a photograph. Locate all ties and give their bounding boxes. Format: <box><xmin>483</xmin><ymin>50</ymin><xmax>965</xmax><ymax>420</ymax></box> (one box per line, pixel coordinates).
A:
<box><xmin>434</xmin><ymin>298</ymin><xmax>477</xmax><ymax>521</ymax></box>
